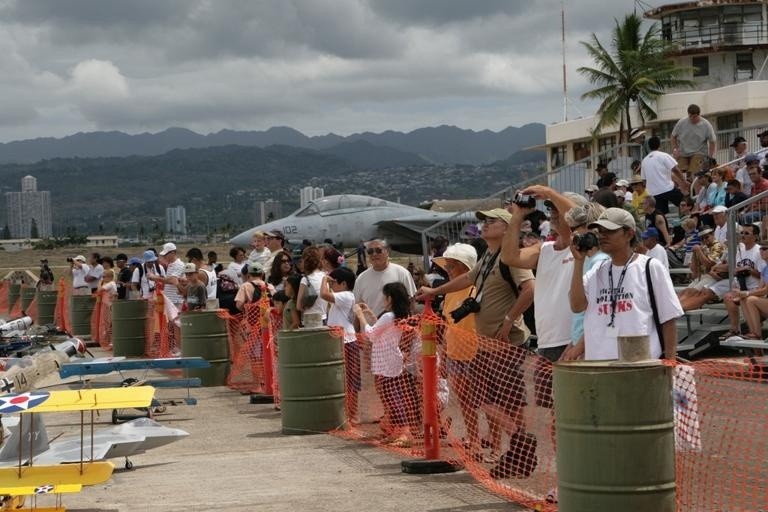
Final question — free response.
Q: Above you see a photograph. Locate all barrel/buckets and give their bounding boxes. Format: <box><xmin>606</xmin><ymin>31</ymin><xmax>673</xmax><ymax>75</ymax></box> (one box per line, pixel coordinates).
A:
<box><xmin>71</xmin><ymin>295</ymin><xmax>97</xmax><ymax>335</ymax></box>
<box><xmin>552</xmin><ymin>359</ymin><xmax>676</xmax><ymax>512</ymax></box>
<box><xmin>179</xmin><ymin>309</ymin><xmax>233</xmax><ymax>387</ymax></box>
<box><xmin>20</xmin><ymin>288</ymin><xmax>36</xmax><ymax>316</ymax></box>
<box><xmin>9</xmin><ymin>284</ymin><xmax>22</xmax><ymax>316</ymax></box>
<box><xmin>277</xmin><ymin>327</ymin><xmax>349</xmax><ymax>436</ymax></box>
<box><xmin>37</xmin><ymin>291</ymin><xmax>58</xmax><ymax>326</ymax></box>
<box><xmin>112</xmin><ymin>298</ymin><xmax>148</xmax><ymax>358</ymax></box>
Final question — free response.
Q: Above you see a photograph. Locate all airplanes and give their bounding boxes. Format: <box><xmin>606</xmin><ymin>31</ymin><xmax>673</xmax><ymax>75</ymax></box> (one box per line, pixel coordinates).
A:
<box><xmin>224</xmin><ymin>156</ymin><xmax>633</xmax><ymax>284</ymax></box>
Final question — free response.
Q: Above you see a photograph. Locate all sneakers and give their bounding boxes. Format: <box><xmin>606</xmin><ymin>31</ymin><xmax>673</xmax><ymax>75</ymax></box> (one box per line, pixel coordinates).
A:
<box><xmin>347</xmin><ymin>415</ymin><xmax>558</xmax><ymax>503</ymax></box>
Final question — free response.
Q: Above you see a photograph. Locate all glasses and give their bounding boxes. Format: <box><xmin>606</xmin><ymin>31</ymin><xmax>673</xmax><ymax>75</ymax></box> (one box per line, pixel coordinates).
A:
<box><xmin>700</xmin><ymin>236</ymin><xmax>709</xmax><ymax>241</ymax></box>
<box><xmin>444</xmin><ymin>264</ymin><xmax>454</xmax><ymax>269</ymax></box>
<box><xmin>367</xmin><ymin>247</ymin><xmax>382</xmax><ymax>255</ymax></box>
<box><xmin>680</xmin><ymin>204</ymin><xmax>685</xmax><ymax>207</ymax></box>
<box><xmin>281</xmin><ymin>260</ymin><xmax>291</xmax><ymax>263</ymax></box>
<box><xmin>741</xmin><ymin>231</ymin><xmax>749</xmax><ymax>235</ymax></box>
<box><xmin>759</xmin><ymin>247</ymin><xmax>768</xmax><ymax>250</ymax></box>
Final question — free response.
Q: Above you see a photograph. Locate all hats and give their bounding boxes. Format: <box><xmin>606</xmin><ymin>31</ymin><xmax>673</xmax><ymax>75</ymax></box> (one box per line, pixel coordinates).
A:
<box><xmin>712</xmin><ymin>206</ymin><xmax>727</xmax><ymax>212</ymax></box>
<box><xmin>587</xmin><ymin>207</ymin><xmax>636</xmax><ymax>231</ymax></box>
<box><xmin>184</xmin><ymin>263</ymin><xmax>199</xmax><ymax>273</ymax></box>
<box><xmin>697</xmin><ymin>225</ymin><xmax>714</xmax><ymax>237</ymax></box>
<box><xmin>160</xmin><ymin>242</ymin><xmax>176</xmax><ymax>255</ymax></box>
<box><xmin>544</xmin><ymin>192</ymin><xmax>587</xmax><ymax>210</ymax></box>
<box><xmin>595</xmin><ymin>164</ymin><xmax>607</xmax><ymax>170</ymax></box>
<box><xmin>126</xmin><ymin>257</ymin><xmax>142</xmax><ymax>265</ymax></box>
<box><xmin>629</xmin><ymin>175</ymin><xmax>646</xmax><ymax>183</ymax></box>
<box><xmin>757</xmin><ymin>130</ymin><xmax>768</xmax><ymax>137</ymax></box>
<box><xmin>113</xmin><ymin>253</ymin><xmax>127</xmax><ymax>260</ymax></box>
<box><xmin>475</xmin><ymin>208</ymin><xmax>512</xmax><ymax>224</ymax></box>
<box><xmin>641</xmin><ymin>228</ymin><xmax>658</xmax><ymax>237</ymax></box>
<box><xmin>144</xmin><ymin>250</ymin><xmax>158</xmax><ymax>262</ymax></box>
<box><xmin>432</xmin><ymin>242</ymin><xmax>477</xmax><ymax>272</ymax></box>
<box><xmin>585</xmin><ymin>185</ymin><xmax>599</xmax><ymax>193</ymax></box>
<box><xmin>615</xmin><ymin>180</ymin><xmax>629</xmax><ymax>187</ymax></box>
<box><xmin>730</xmin><ymin>137</ymin><xmax>747</xmax><ymax>147</ymax></box>
<box><xmin>248</xmin><ymin>262</ymin><xmax>263</xmax><ymax>273</ymax></box>
<box><xmin>73</xmin><ymin>255</ymin><xmax>86</xmax><ymax>262</ymax></box>
<box><xmin>263</xmin><ymin>229</ymin><xmax>284</xmax><ymax>237</ymax></box>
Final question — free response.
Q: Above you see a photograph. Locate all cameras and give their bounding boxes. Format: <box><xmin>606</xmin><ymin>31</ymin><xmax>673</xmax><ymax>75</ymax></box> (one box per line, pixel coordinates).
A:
<box><xmin>449</xmin><ymin>298</ymin><xmax>480</xmax><ymax>323</ymax></box>
<box><xmin>513</xmin><ymin>193</ymin><xmax>536</xmax><ymax>209</ymax></box>
<box><xmin>735</xmin><ymin>270</ymin><xmax>751</xmax><ymax>291</ymax></box>
<box><xmin>573</xmin><ymin>232</ymin><xmax>599</xmax><ymax>251</ymax></box>
<box><xmin>67</xmin><ymin>257</ymin><xmax>73</xmax><ymax>262</ymax></box>
<box><xmin>149</xmin><ymin>287</ymin><xmax>156</xmax><ymax>292</ymax></box>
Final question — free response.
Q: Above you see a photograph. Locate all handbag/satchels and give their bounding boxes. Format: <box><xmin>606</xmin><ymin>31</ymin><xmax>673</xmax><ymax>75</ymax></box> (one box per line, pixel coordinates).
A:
<box><xmin>301</xmin><ymin>281</ymin><xmax>318</xmax><ymax>308</ymax></box>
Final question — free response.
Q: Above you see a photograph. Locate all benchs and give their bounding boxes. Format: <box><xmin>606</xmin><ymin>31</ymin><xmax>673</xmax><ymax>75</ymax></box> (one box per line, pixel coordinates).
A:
<box><xmin>662</xmin><ymin>267</ymin><xmax>768</xmax><ymax>371</ymax></box>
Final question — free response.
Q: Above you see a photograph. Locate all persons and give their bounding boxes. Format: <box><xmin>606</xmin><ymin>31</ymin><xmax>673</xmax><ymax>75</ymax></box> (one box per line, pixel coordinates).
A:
<box><xmin>415</xmin><ymin>100</ymin><xmax>767</xmax><ymax>505</ymax></box>
<box><xmin>34</xmin><ymin>229</ymin><xmax>444</xmax><ymax>449</ymax></box>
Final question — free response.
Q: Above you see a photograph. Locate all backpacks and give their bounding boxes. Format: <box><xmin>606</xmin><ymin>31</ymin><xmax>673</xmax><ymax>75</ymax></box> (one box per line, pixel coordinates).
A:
<box><xmin>247</xmin><ymin>282</ymin><xmax>275</xmax><ymax>326</ymax></box>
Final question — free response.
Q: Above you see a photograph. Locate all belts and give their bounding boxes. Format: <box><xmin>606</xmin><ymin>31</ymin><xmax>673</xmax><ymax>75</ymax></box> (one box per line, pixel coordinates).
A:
<box><xmin>75</xmin><ymin>286</ymin><xmax>89</xmax><ymax>290</ymax></box>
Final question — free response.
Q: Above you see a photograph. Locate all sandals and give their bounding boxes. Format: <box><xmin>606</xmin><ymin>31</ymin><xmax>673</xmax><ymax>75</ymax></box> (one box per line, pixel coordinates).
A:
<box><xmin>719</xmin><ymin>329</ymin><xmax>763</xmax><ymax>340</ymax></box>
